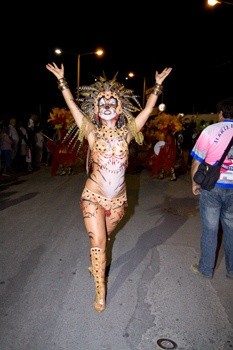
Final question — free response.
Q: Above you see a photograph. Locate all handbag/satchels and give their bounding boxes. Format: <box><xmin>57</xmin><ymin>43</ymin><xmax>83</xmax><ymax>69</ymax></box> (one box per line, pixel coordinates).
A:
<box><xmin>193</xmin><ymin>162</ymin><xmax>221</xmax><ymax>192</ymax></box>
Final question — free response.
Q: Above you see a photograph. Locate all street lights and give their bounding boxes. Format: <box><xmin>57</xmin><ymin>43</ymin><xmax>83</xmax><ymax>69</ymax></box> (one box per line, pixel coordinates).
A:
<box><xmin>54</xmin><ymin>47</ymin><xmax>105</xmax><ymax>102</ymax></box>
<box><xmin>205</xmin><ymin>0</ymin><xmax>233</xmax><ymax>8</ymax></box>
<box><xmin>128</xmin><ymin>72</ymin><xmax>147</xmax><ymax>106</ymax></box>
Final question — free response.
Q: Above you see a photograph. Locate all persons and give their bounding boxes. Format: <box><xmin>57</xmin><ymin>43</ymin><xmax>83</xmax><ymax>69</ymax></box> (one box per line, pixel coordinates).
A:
<box><xmin>190</xmin><ymin>99</ymin><xmax>233</xmax><ymax>279</ymax></box>
<box><xmin>0</xmin><ymin>115</ymin><xmax>44</xmax><ymax>184</ymax></box>
<box><xmin>47</xmin><ymin>106</ymin><xmax>215</xmax><ymax>181</ymax></box>
<box><xmin>46</xmin><ymin>62</ymin><xmax>172</xmax><ymax>312</ymax></box>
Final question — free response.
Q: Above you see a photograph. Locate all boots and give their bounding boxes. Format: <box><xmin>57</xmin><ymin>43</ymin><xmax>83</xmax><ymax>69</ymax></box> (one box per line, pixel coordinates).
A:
<box><xmin>90</xmin><ymin>248</ymin><xmax>108</xmax><ymax>313</ymax></box>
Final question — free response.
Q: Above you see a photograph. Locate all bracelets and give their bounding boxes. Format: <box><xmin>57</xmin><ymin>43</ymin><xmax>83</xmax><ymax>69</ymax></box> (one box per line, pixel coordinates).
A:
<box><xmin>152</xmin><ymin>83</ymin><xmax>163</xmax><ymax>95</ymax></box>
<box><xmin>58</xmin><ymin>78</ymin><xmax>69</xmax><ymax>91</ymax></box>
